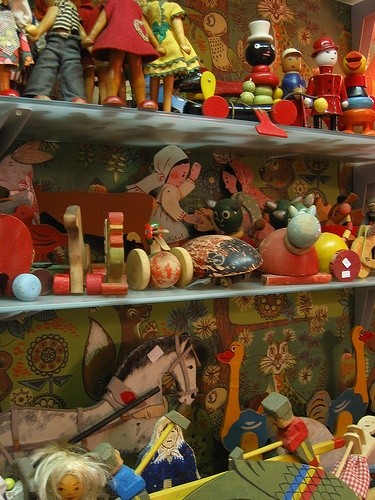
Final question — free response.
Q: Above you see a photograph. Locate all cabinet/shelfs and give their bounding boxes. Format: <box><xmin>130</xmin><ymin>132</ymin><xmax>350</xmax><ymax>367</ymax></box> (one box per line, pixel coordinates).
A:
<box><xmin>0</xmin><ymin>95</ymin><xmax>375</xmax><ymax>313</ymax></box>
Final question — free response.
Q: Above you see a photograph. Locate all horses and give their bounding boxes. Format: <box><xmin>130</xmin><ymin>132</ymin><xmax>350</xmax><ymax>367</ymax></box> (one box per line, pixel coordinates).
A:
<box><xmin>0</xmin><ymin>332</ymin><xmax>208</xmax><ymax>476</ymax></box>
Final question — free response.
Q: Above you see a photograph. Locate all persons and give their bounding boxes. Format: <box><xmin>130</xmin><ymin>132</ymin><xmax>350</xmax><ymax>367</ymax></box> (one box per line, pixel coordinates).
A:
<box><xmin>0</xmin><ymin>0</ymin><xmax>375</xmax><ymax>138</ymax></box>
<box><xmin>0</xmin><ymin>136</ymin><xmax>375</xmax><ymax>252</ymax></box>
<box><xmin>34</xmin><ymin>393</ymin><xmax>373</xmax><ymax>500</ymax></box>
